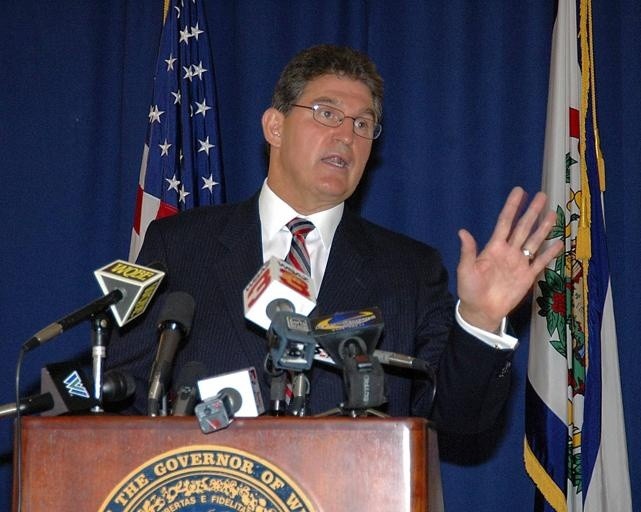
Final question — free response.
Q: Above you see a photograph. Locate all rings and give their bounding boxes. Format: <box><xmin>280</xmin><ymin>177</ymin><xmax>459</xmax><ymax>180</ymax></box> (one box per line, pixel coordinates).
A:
<box><xmin>522</xmin><ymin>246</ymin><xmax>539</xmax><ymax>260</ymax></box>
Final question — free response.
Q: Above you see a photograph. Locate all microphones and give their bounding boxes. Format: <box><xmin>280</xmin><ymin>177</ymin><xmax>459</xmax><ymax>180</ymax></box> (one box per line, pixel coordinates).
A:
<box><xmin>173</xmin><ymin>362</ymin><xmax>209</xmax><ymax>417</ymax></box>
<box><xmin>148</xmin><ymin>292</ymin><xmax>195</xmax><ymax>417</ymax></box>
<box><xmin>311</xmin><ymin>306</ymin><xmax>388</xmax><ymax>409</ymax></box>
<box><xmin>314</xmin><ymin>335</ymin><xmax>434</xmax><ymax>376</ymax></box>
<box><xmin>21</xmin><ymin>260</ymin><xmax>167</xmax><ymax>353</ymax></box>
<box><xmin>243</xmin><ymin>256</ymin><xmax>318</xmax><ymax>371</ymax></box>
<box><xmin>194</xmin><ymin>366</ymin><xmax>264</xmax><ymax>434</ymax></box>
<box><xmin>0</xmin><ymin>359</ymin><xmax>136</xmax><ymax>419</ymax></box>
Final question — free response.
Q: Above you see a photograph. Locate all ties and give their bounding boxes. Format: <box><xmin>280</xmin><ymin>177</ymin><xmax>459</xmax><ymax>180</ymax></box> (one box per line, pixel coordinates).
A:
<box><xmin>281</xmin><ymin>216</ymin><xmax>314</xmax><ymax>281</ymax></box>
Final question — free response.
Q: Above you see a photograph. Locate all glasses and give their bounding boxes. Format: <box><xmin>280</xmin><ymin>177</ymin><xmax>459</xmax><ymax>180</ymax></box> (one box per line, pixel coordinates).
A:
<box><xmin>278</xmin><ymin>100</ymin><xmax>383</xmax><ymax>141</ymax></box>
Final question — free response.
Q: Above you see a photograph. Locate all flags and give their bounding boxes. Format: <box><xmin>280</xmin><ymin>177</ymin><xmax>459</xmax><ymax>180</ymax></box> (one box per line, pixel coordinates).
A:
<box><xmin>518</xmin><ymin>0</ymin><xmax>638</xmax><ymax>510</ymax></box>
<box><xmin>125</xmin><ymin>0</ymin><xmax>228</xmax><ymax>266</ymax></box>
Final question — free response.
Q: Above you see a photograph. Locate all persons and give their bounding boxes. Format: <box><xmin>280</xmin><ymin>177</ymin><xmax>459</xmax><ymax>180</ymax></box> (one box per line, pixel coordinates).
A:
<box><xmin>77</xmin><ymin>42</ymin><xmax>567</xmax><ymax>471</ymax></box>
